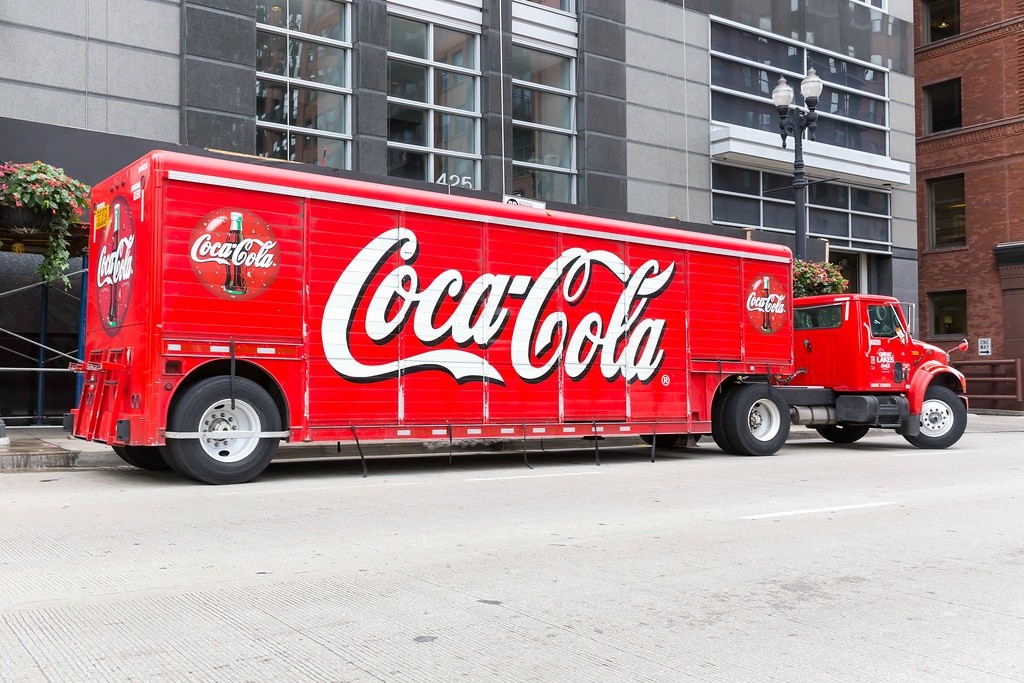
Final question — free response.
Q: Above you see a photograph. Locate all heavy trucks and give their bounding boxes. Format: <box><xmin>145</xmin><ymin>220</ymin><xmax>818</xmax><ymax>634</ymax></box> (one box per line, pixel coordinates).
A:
<box><xmin>63</xmin><ymin>148</ymin><xmax>970</xmax><ymax>487</ymax></box>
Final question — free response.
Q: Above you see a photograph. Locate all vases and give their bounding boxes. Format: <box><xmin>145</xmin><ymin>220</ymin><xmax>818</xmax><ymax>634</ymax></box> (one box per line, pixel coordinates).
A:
<box><xmin>0</xmin><ymin>205</ymin><xmax>54</xmax><ymax>237</ymax></box>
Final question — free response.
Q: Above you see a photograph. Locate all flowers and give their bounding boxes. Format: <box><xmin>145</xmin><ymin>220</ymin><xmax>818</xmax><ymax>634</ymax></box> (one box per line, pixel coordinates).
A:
<box><xmin>791</xmin><ymin>257</ymin><xmax>849</xmax><ymax>296</ymax></box>
<box><xmin>0</xmin><ymin>160</ymin><xmax>93</xmax><ymax>290</ymax></box>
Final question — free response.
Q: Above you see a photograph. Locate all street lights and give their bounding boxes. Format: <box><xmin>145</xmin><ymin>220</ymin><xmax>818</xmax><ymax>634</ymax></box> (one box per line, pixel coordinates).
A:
<box><xmin>773</xmin><ymin>67</ymin><xmax>824</xmax><ymax>261</ymax></box>
